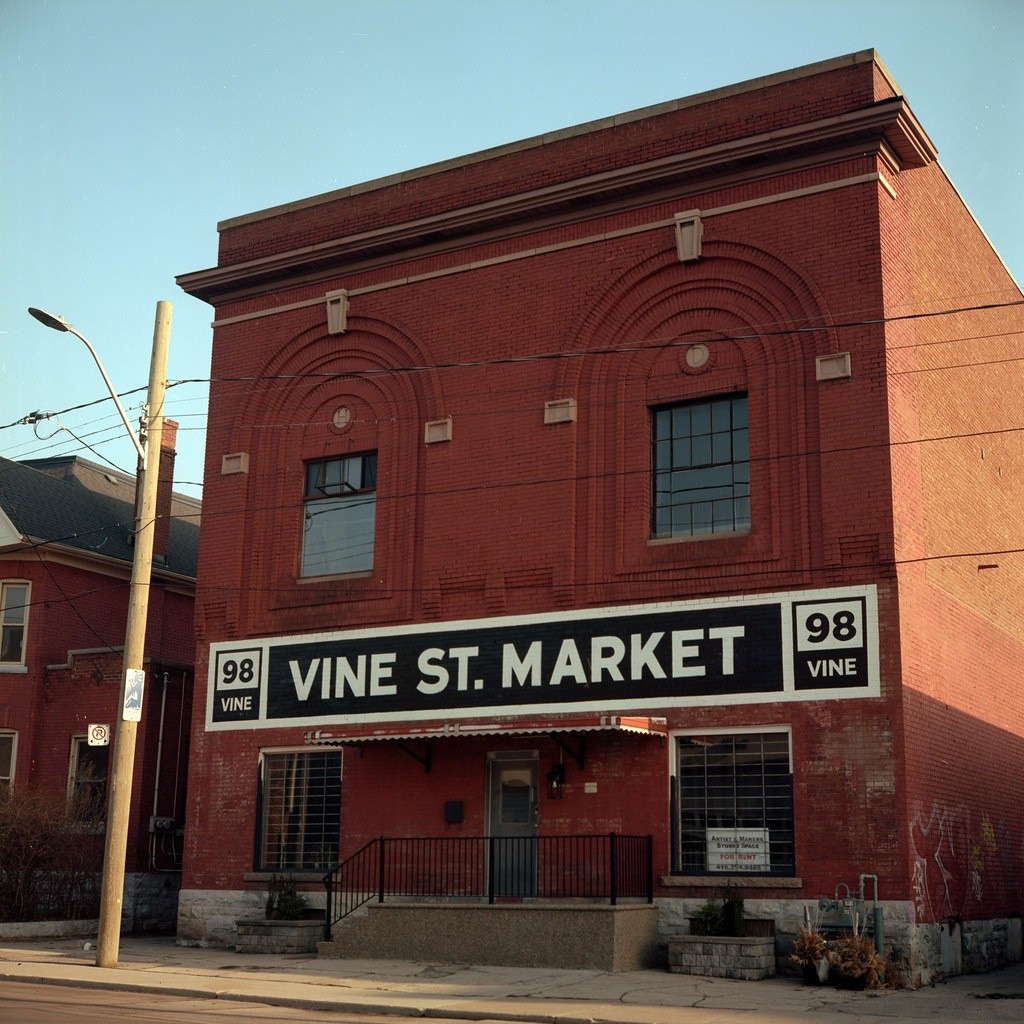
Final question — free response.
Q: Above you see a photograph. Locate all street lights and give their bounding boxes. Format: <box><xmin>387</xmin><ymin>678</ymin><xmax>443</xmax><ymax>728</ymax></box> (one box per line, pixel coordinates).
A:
<box><xmin>26</xmin><ymin>301</ymin><xmax>174</xmax><ymax>970</ymax></box>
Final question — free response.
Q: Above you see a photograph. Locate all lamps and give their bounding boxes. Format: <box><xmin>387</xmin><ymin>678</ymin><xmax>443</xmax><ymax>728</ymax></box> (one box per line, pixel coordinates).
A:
<box><xmin>545</xmin><ymin>764</ymin><xmax>568</xmax><ymax>800</ymax></box>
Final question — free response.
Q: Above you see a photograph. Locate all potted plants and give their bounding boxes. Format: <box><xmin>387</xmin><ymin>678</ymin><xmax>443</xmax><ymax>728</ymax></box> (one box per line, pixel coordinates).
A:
<box><xmin>792</xmin><ymin>903</ymin><xmax>829</xmax><ymax>984</ymax></box>
<box><xmin>684</xmin><ymin>882</ymin><xmax>775</xmax><ymax>936</ymax></box>
<box><xmin>265</xmin><ymin>873</ymin><xmax>327</xmax><ymax>921</ymax></box>
<box><xmin>826</xmin><ymin>911</ymin><xmax>873</xmax><ymax>989</ymax></box>
